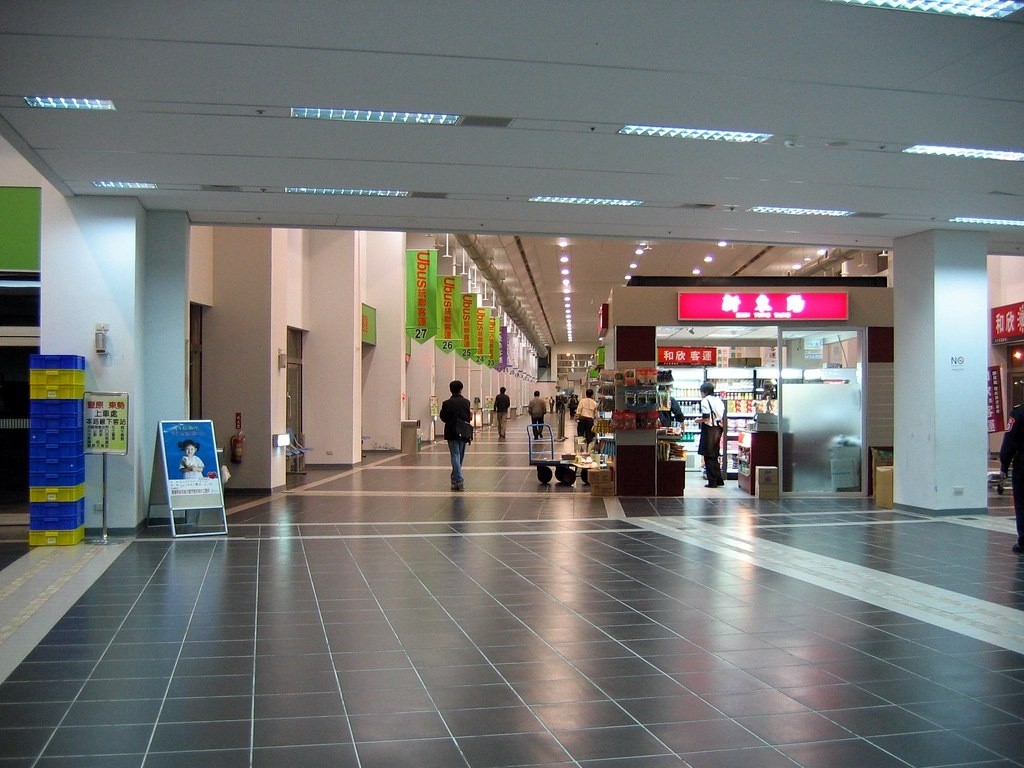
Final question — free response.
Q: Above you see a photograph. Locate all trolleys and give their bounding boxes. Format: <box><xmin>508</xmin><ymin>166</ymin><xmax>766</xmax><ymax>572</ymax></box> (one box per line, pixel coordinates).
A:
<box><xmin>526</xmin><ymin>424</ymin><xmax>609</xmax><ymax>487</ymax></box>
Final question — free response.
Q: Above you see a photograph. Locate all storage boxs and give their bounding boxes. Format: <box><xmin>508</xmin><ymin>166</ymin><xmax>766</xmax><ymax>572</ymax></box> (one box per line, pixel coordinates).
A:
<box><xmin>834</xmin><ymin>445</ymin><xmax>860</xmax><ymax>463</ymax></box>
<box><xmin>755</xmin><ymin>482</ymin><xmax>779</xmax><ymax>499</ymax></box>
<box><xmin>599</xmin><ymin>469</ymin><xmax>611</xmax><ymax>484</ymax></box>
<box><xmin>831</xmin><ymin>458</ymin><xmax>859</xmax><ymax>488</ymax></box>
<box><xmin>587</xmin><ymin>469</ymin><xmax>599</xmax><ymax>484</ymax></box>
<box><xmin>590</xmin><ymin>481</ymin><xmax>614</xmax><ymax>496</ymax></box>
<box><xmin>755</xmin><ymin>466</ymin><xmax>778</xmax><ymax>484</ymax></box>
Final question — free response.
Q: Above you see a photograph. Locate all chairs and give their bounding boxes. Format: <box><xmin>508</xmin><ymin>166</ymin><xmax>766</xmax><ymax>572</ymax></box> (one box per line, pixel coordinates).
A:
<box><xmin>361</xmin><ymin>435</ymin><xmax>372</xmax><ymax>457</ymax></box>
<box><xmin>286</xmin><ymin>428</ymin><xmax>314</xmax><ymax>475</ymax></box>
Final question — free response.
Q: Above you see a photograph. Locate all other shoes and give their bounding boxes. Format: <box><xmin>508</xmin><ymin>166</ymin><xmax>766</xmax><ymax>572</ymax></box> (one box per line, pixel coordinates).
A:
<box><xmin>704</xmin><ymin>484</ymin><xmax>716</xmax><ymax>487</ymax></box>
<box><xmin>715</xmin><ymin>481</ymin><xmax>724</xmax><ymax>486</ymax></box>
<box><xmin>450</xmin><ymin>478</ymin><xmax>457</xmax><ymax>490</ymax></box>
<box><xmin>457</xmin><ymin>480</ymin><xmax>465</xmax><ymax>491</ymax></box>
<box><xmin>1012</xmin><ymin>544</ymin><xmax>1023</xmax><ymax>553</ymax></box>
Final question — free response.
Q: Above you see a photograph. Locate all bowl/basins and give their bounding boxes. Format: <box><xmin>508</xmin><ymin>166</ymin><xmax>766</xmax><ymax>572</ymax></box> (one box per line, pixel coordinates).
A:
<box><xmin>561</xmin><ymin>455</ymin><xmax>576</xmax><ymax>460</ymax></box>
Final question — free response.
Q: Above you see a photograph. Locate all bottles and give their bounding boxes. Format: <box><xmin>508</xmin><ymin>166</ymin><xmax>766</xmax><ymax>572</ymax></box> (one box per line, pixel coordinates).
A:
<box><xmin>678</xmin><ymin>401</ymin><xmax>700</xmax><ymax>415</ymax></box>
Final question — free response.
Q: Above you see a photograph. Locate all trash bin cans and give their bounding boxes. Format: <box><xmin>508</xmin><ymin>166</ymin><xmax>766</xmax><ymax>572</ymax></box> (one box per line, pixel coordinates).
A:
<box><xmin>522</xmin><ymin>405</ymin><xmax>529</xmax><ymax>416</ymax></box>
<box><xmin>510</xmin><ymin>406</ymin><xmax>517</xmax><ymax>420</ymax></box>
<box><xmin>491</xmin><ymin>409</ymin><xmax>498</xmax><ymax>427</ymax></box>
<box><xmin>400</xmin><ymin>420</ymin><xmax>422</xmax><ymax>453</ymax></box>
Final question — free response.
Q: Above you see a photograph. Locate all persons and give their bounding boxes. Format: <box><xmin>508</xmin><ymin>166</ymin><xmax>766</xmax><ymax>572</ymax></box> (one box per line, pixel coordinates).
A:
<box><xmin>439</xmin><ymin>381</ymin><xmax>471</xmax><ymax>490</ymax></box>
<box><xmin>657</xmin><ymin>384</ymin><xmax>686</xmax><ymax>430</ymax></box>
<box><xmin>575</xmin><ymin>389</ymin><xmax>600</xmax><ymax>444</ymax></box>
<box><xmin>762</xmin><ymin>379</ymin><xmax>776</xmax><ymax>399</ymax></box>
<box><xmin>178</xmin><ymin>440</ymin><xmax>205</xmax><ymax>478</ymax></box>
<box><xmin>695</xmin><ymin>382</ymin><xmax>726</xmax><ymax>488</ymax></box>
<box><xmin>494</xmin><ymin>386</ymin><xmax>510</xmax><ymax>438</ymax></box>
<box><xmin>549</xmin><ymin>396</ymin><xmax>554</xmax><ymax>414</ymax></box>
<box><xmin>528</xmin><ymin>391</ymin><xmax>547</xmax><ymax>440</ymax></box>
<box><xmin>999</xmin><ymin>405</ymin><xmax>1024</xmax><ymax>553</ymax></box>
<box><xmin>556</xmin><ymin>394</ymin><xmax>579</xmax><ymax>418</ymax></box>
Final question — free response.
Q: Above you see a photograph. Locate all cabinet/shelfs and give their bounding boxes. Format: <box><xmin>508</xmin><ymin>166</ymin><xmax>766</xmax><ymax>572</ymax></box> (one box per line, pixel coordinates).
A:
<box><xmin>657</xmin><ymin>368</ymin><xmax>849</xmax><ymax>497</ymax></box>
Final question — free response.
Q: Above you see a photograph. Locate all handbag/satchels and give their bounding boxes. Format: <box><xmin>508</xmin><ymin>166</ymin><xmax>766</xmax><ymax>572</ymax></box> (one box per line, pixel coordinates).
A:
<box><xmin>457</xmin><ymin>419</ymin><xmax>473</xmax><ymax>446</ymax></box>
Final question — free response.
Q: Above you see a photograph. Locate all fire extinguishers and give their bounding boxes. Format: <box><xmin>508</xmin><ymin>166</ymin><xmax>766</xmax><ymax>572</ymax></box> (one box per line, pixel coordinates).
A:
<box><xmin>230</xmin><ymin>431</ymin><xmax>246</xmax><ymax>464</ymax></box>
